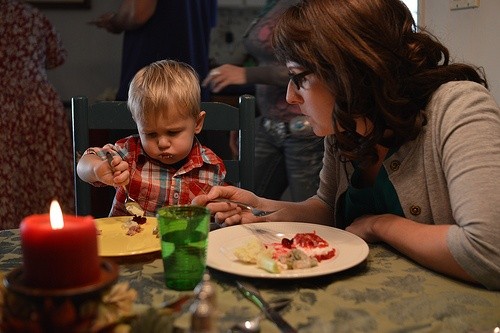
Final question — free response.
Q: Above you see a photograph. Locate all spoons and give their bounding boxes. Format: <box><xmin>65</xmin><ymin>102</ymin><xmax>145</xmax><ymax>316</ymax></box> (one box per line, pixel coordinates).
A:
<box><xmin>227</xmin><ymin>298</ymin><xmax>292</xmax><ymax>333</ymax></box>
<box><xmin>107</xmin><ymin>151</ymin><xmax>144</xmax><ymax>216</ymax></box>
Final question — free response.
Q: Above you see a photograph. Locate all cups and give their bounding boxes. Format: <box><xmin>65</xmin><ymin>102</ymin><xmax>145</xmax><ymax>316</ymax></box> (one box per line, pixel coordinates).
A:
<box><xmin>156</xmin><ymin>204</ymin><xmax>210</xmax><ymax>291</ymax></box>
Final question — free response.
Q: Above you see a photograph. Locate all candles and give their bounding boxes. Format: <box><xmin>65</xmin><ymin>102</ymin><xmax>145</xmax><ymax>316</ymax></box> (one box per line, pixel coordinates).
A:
<box><xmin>20</xmin><ymin>199</ymin><xmax>100</xmax><ymax>289</ymax></box>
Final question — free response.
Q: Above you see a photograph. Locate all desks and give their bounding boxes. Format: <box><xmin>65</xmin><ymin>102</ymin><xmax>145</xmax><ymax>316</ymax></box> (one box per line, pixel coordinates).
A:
<box><xmin>0</xmin><ymin>228</ymin><xmax>500</xmax><ymax>332</ymax></box>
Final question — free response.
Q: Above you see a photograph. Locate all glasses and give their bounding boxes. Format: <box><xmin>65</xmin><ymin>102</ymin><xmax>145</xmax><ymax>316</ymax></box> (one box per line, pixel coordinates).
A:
<box><xmin>289</xmin><ymin>70</ymin><xmax>310</xmax><ymax>91</ymax></box>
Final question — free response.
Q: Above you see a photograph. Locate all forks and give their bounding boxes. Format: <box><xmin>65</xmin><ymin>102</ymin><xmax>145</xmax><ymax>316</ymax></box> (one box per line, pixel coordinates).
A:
<box><xmin>210</xmin><ymin>198</ymin><xmax>286</xmax><ymax>218</ymax></box>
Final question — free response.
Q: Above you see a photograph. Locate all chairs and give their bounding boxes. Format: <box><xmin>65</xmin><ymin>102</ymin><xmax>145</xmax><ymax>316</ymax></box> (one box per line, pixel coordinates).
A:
<box><xmin>71</xmin><ymin>93</ymin><xmax>255</xmax><ymax>218</ymax></box>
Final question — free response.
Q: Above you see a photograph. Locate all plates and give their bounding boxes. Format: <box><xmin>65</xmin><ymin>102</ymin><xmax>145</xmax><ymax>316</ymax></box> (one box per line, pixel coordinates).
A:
<box><xmin>92</xmin><ymin>215</ymin><xmax>161</xmax><ymax>257</ymax></box>
<box><xmin>204</xmin><ymin>222</ymin><xmax>370</xmax><ymax>280</ymax></box>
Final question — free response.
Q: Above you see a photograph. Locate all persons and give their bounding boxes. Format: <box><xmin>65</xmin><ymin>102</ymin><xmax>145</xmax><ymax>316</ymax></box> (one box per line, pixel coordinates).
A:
<box><xmin>77</xmin><ymin>60</ymin><xmax>225</xmax><ymax>217</ymax></box>
<box><xmin>0</xmin><ymin>0</ymin><xmax>76</xmax><ymax>229</ymax></box>
<box><xmin>96</xmin><ymin>0</ymin><xmax>217</xmax><ymax>101</ymax></box>
<box><xmin>191</xmin><ymin>0</ymin><xmax>500</xmax><ymax>291</ymax></box>
<box><xmin>203</xmin><ymin>0</ymin><xmax>324</xmax><ymax>202</ymax></box>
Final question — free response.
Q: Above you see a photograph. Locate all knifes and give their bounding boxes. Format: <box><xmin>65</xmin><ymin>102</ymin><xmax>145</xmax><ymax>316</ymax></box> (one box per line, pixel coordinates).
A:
<box><xmin>236</xmin><ymin>280</ymin><xmax>298</xmax><ymax>333</ymax></box>
